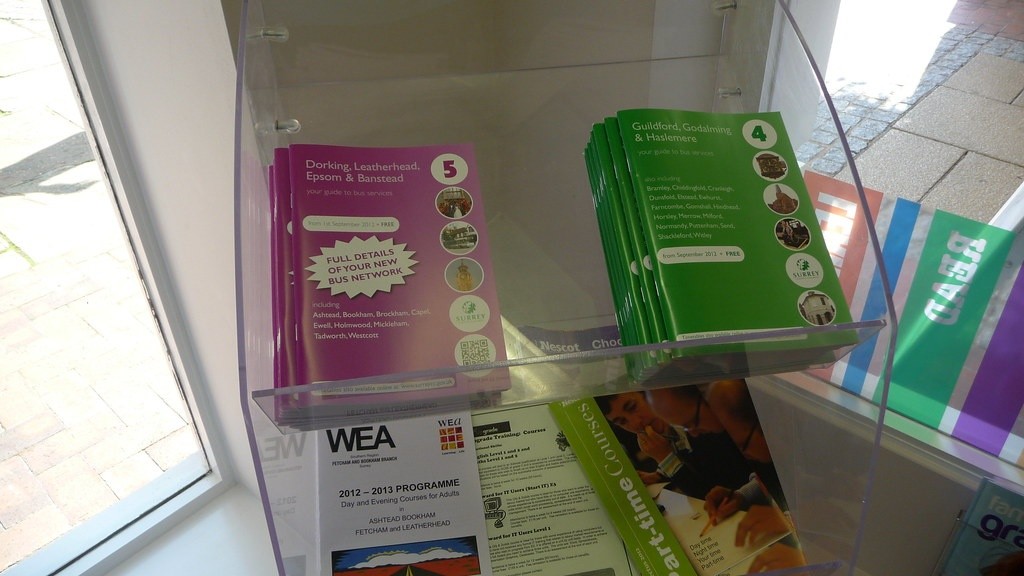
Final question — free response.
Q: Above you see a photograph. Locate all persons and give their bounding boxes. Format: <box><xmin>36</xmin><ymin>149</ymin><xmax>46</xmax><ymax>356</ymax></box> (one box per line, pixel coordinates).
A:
<box><xmin>639</xmin><ymin>367</ymin><xmax>772</xmax><ymax>507</ymax></box>
<box><xmin>703</xmin><ymin>378</ymin><xmax>787</xmax><ymax>548</ymax></box>
<box><xmin>595</xmin><ymin>377</ymin><xmax>700</xmax><ymax>499</ymax></box>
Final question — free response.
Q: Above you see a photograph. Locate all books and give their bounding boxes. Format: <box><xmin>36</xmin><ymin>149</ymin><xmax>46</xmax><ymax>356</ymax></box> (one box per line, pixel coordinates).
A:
<box><xmin>547</xmin><ymin>376</ymin><xmax>814</xmax><ymax>576</ymax></box>
<box><xmin>584</xmin><ymin>109</ymin><xmax>861</xmax><ymax>387</ymax></box>
<box><xmin>313</xmin><ymin>399</ymin><xmax>493</xmax><ymax>576</ymax></box>
<box><xmin>775</xmin><ymin>169</ymin><xmax>1024</xmax><ymax>495</ymax></box>
<box><xmin>936</xmin><ymin>475</ymin><xmax>1024</xmax><ymax>576</ymax></box>
<box><xmin>268</xmin><ymin>141</ymin><xmax>510</xmax><ymax>431</ymax></box>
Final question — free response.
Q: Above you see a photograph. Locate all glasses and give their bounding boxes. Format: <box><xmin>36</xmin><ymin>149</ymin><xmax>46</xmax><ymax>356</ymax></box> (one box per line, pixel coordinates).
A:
<box><xmin>672</xmin><ymin>398</ymin><xmax>701</xmax><ymax>432</ymax></box>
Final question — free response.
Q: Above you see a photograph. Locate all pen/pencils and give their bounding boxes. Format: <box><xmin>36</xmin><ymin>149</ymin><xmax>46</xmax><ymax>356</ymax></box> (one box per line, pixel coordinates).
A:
<box><xmin>638</xmin><ymin>431</ymin><xmax>680</xmax><ymax>439</ymax></box>
<box><xmin>699</xmin><ymin>489</ymin><xmax>735</xmax><ymax>537</ymax></box>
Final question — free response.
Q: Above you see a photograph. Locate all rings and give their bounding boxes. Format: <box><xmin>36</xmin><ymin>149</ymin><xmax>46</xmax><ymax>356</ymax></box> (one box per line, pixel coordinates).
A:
<box><xmin>641</xmin><ymin>441</ymin><xmax>646</xmax><ymax>445</ymax></box>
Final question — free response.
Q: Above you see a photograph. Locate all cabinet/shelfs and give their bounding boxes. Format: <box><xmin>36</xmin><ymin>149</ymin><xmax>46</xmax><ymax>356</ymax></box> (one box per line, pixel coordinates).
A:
<box><xmin>222</xmin><ymin>1</ymin><xmax>897</xmax><ymax>576</ymax></box>
